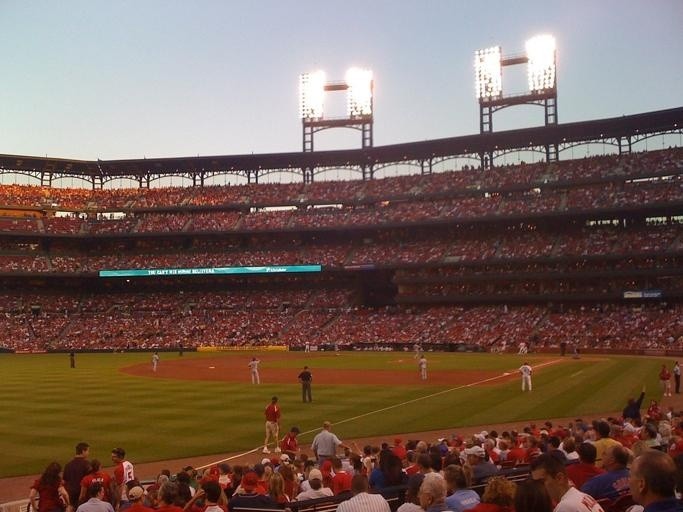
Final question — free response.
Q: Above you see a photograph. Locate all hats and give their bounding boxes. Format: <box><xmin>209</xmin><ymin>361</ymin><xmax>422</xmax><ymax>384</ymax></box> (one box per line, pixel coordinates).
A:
<box><xmin>108</xmin><ymin>413</ymin><xmax>625</xmax><ymax>504</ymax></box>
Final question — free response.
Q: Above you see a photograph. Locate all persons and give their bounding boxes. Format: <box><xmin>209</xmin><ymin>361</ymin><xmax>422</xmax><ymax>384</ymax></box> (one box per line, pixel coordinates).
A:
<box><xmin>69</xmin><ymin>350</ymin><xmax>74</xmax><ymax>368</ymax></box>
<box><xmin>658</xmin><ymin>364</ymin><xmax>673</xmax><ymax>396</ymax></box>
<box><xmin>297</xmin><ymin>365</ymin><xmax>313</xmax><ymax>403</ymax></box>
<box><xmin>1</xmin><ymin>146</ymin><xmax>682</xmax><ymax>360</ymax></box>
<box><xmin>247</xmin><ymin>356</ymin><xmax>260</xmax><ymax>385</ymax></box>
<box><xmin>151</xmin><ymin>352</ymin><xmax>159</xmax><ymax>371</ymax></box>
<box><xmin>24</xmin><ymin>395</ymin><xmax>683</xmax><ymax>512</ymax></box>
<box><xmin>418</xmin><ymin>355</ymin><xmax>428</xmax><ymax>380</ymax></box>
<box><xmin>518</xmin><ymin>362</ymin><xmax>533</xmax><ymax>393</ymax></box>
<box><xmin>673</xmin><ymin>361</ymin><xmax>681</xmax><ymax>393</ymax></box>
<box><xmin>620</xmin><ymin>385</ymin><xmax>646</xmax><ymax>423</ymax></box>
